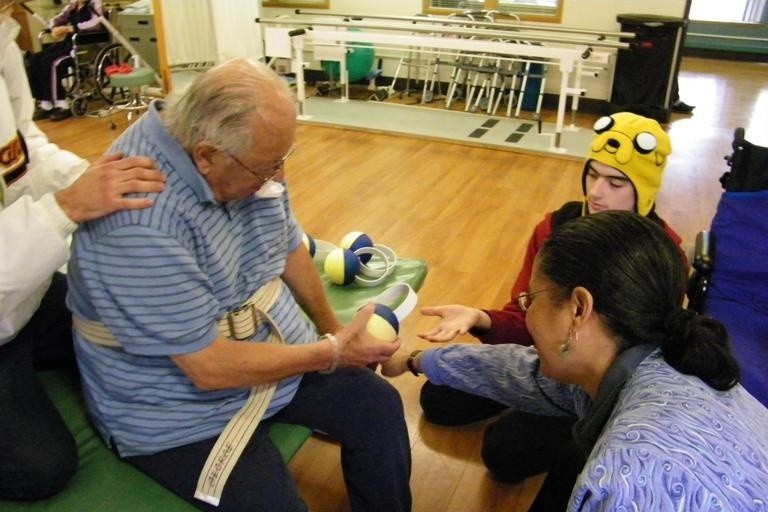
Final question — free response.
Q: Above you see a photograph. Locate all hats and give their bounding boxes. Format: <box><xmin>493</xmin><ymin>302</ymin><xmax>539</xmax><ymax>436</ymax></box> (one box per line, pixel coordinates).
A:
<box><xmin>581</xmin><ymin>112</ymin><xmax>671</xmax><ymax>218</ymax></box>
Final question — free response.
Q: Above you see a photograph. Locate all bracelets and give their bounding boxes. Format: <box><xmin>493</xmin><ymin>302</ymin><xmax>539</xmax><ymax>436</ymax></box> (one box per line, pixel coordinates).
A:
<box><xmin>318</xmin><ymin>334</ymin><xmax>339</xmax><ymax>373</ymax></box>
<box><xmin>407</xmin><ymin>349</ymin><xmax>422</xmax><ymax>377</ymax></box>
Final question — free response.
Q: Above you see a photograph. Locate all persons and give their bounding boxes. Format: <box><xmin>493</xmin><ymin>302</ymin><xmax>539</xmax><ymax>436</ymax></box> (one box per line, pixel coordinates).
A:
<box><xmin>417</xmin><ymin>111</ymin><xmax>688</xmax><ymax>481</ymax></box>
<box><xmin>381</xmin><ymin>210</ymin><xmax>767</xmax><ymax>512</ymax></box>
<box><xmin>0</xmin><ymin>14</ymin><xmax>167</xmax><ymax>505</ymax></box>
<box><xmin>30</xmin><ymin>2</ymin><xmax>103</xmax><ymax>111</ymax></box>
<box><xmin>64</xmin><ymin>57</ymin><xmax>412</xmax><ymax>512</ymax></box>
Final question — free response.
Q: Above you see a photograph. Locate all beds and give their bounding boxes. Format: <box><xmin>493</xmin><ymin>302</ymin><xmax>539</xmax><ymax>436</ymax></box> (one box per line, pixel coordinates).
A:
<box><xmin>6</xmin><ymin>226</ymin><xmax>428</xmax><ymax>512</ymax></box>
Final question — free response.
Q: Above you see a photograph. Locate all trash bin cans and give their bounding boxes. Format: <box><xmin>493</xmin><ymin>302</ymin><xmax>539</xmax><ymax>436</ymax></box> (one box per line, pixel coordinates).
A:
<box><xmin>609</xmin><ymin>13</ymin><xmax>688</xmax><ymax>123</ymax></box>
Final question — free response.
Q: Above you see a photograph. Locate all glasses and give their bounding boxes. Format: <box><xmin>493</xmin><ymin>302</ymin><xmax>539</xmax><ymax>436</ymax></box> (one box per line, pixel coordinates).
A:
<box><xmin>516</xmin><ymin>285</ymin><xmax>566</xmax><ymax>310</ymax></box>
<box><xmin>223</xmin><ymin>143</ymin><xmax>295</xmax><ymax>181</ymax></box>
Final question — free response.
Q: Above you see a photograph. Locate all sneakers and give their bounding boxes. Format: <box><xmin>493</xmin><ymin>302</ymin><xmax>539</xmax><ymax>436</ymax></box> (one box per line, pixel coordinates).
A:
<box><xmin>34</xmin><ymin>106</ymin><xmax>71</xmax><ymax>122</ymax></box>
